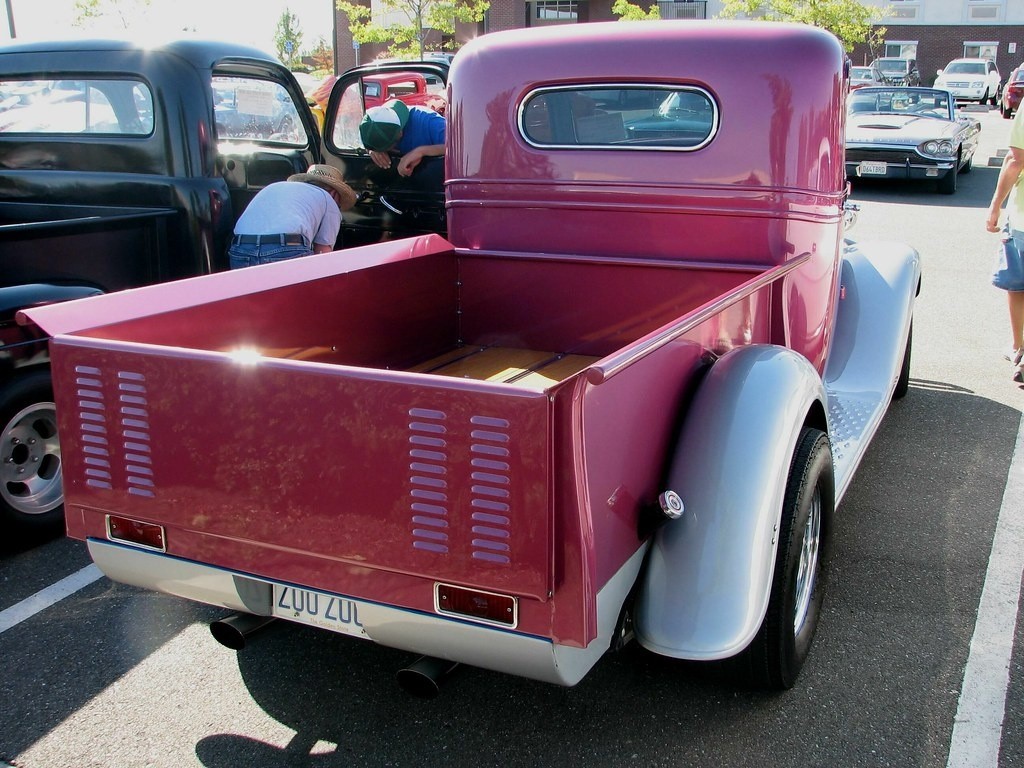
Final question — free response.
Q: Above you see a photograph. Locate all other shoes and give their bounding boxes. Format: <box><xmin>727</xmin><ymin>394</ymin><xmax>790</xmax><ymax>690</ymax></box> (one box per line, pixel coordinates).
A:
<box><xmin>1013</xmin><ymin>347</ymin><xmax>1024</xmax><ymax>363</ymax></box>
<box><xmin>1013</xmin><ymin>355</ymin><xmax>1024</xmax><ymax>382</ymax></box>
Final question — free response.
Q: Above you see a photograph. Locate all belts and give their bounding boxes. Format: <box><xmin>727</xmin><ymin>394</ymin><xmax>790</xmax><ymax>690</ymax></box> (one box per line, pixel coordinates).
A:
<box><xmin>232</xmin><ymin>233</ymin><xmax>303</xmax><ymax>244</ymax></box>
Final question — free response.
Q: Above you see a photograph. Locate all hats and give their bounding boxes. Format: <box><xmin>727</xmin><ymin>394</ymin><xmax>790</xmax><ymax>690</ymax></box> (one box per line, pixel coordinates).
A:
<box><xmin>358</xmin><ymin>99</ymin><xmax>409</xmax><ymax>153</ymax></box>
<box><xmin>286</xmin><ymin>164</ymin><xmax>356</xmax><ymax>211</ymax></box>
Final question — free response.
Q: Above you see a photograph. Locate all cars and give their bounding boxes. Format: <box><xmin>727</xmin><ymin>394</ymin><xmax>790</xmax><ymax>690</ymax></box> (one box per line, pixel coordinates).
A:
<box><xmin>847</xmin><ymin>65</ymin><xmax>898</xmax><ymax>112</ymax></box>
<box><xmin>312</xmin><ymin>53</ymin><xmax>454</xmax><ymax>128</ymax></box>
<box><xmin>526</xmin><ymin>90</ymin><xmax>714</xmax><ymax>144</ymax></box>
<box><xmin>0</xmin><ymin>80</ymin><xmax>325</xmax><ymax>140</ymax></box>
<box><xmin>999</xmin><ymin>62</ymin><xmax>1024</xmax><ymax>118</ymax></box>
<box><xmin>845</xmin><ymin>85</ymin><xmax>982</xmax><ymax>194</ymax></box>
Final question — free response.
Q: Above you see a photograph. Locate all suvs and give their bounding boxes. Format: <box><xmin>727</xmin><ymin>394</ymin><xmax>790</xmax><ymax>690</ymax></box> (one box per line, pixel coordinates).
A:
<box><xmin>933</xmin><ymin>58</ymin><xmax>1003</xmax><ymax>109</ymax></box>
<box><xmin>863</xmin><ymin>57</ymin><xmax>923</xmax><ymax>104</ymax></box>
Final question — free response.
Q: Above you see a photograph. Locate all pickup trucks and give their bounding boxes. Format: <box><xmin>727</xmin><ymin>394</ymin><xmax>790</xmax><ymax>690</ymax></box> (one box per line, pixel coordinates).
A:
<box><xmin>0</xmin><ymin>40</ymin><xmax>451</xmax><ymax>551</ymax></box>
<box><xmin>14</xmin><ymin>10</ymin><xmax>923</xmax><ymax>700</ymax></box>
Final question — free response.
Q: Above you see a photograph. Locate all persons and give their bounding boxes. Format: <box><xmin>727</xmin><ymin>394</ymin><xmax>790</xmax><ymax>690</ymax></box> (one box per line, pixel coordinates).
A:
<box><xmin>359</xmin><ymin>99</ymin><xmax>446</xmax><ymax>178</ymax></box>
<box><xmin>986</xmin><ymin>96</ymin><xmax>1024</xmax><ymax>382</ymax></box>
<box><xmin>227</xmin><ymin>164</ymin><xmax>358</xmax><ymax>270</ymax></box>
